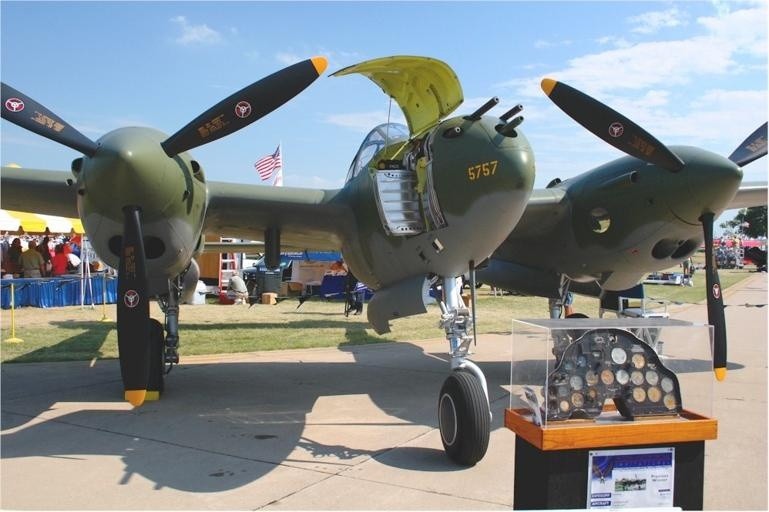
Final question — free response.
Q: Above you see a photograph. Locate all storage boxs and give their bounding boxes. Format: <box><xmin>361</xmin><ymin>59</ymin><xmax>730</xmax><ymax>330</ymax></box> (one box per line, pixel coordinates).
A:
<box><xmin>261</xmin><ymin>292</ymin><xmax>276</xmax><ymax>305</ymax></box>
<box><xmin>280</xmin><ymin>281</ymin><xmax>289</xmax><ymax>297</ymax></box>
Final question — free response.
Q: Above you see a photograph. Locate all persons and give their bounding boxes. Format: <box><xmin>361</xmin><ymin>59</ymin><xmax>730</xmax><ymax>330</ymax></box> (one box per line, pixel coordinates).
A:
<box><xmin>718</xmin><ymin>236</ymin><xmax>725</xmax><ymax>246</ymax></box>
<box><xmin>226</xmin><ymin>269</ymin><xmax>248</xmax><ymax>304</ymax></box>
<box><xmin>716</xmin><ymin>240</ymin><xmax>727</xmax><ymax>269</ymax></box>
<box><xmin>680</xmin><ymin>256</ymin><xmax>695</xmax><ymax>286</ymax></box>
<box><xmin>0</xmin><ymin>231</ymin><xmax>81</xmax><ymax>279</ymax></box>
<box><xmin>732</xmin><ymin>235</ymin><xmax>739</xmax><ymax>246</ymax></box>
<box><xmin>724</xmin><ymin>240</ymin><xmax>740</xmax><ymax>269</ymax></box>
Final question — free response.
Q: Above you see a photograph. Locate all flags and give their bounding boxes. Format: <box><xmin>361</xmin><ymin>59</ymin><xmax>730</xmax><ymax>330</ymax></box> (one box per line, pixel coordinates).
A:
<box><xmin>255</xmin><ymin>144</ymin><xmax>281</xmax><ymax>181</ymax></box>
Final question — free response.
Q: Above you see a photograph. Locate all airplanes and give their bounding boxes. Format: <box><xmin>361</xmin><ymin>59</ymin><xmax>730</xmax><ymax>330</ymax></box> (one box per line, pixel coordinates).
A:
<box><xmin>0</xmin><ymin>55</ymin><xmax>768</xmax><ymax>465</ymax></box>
